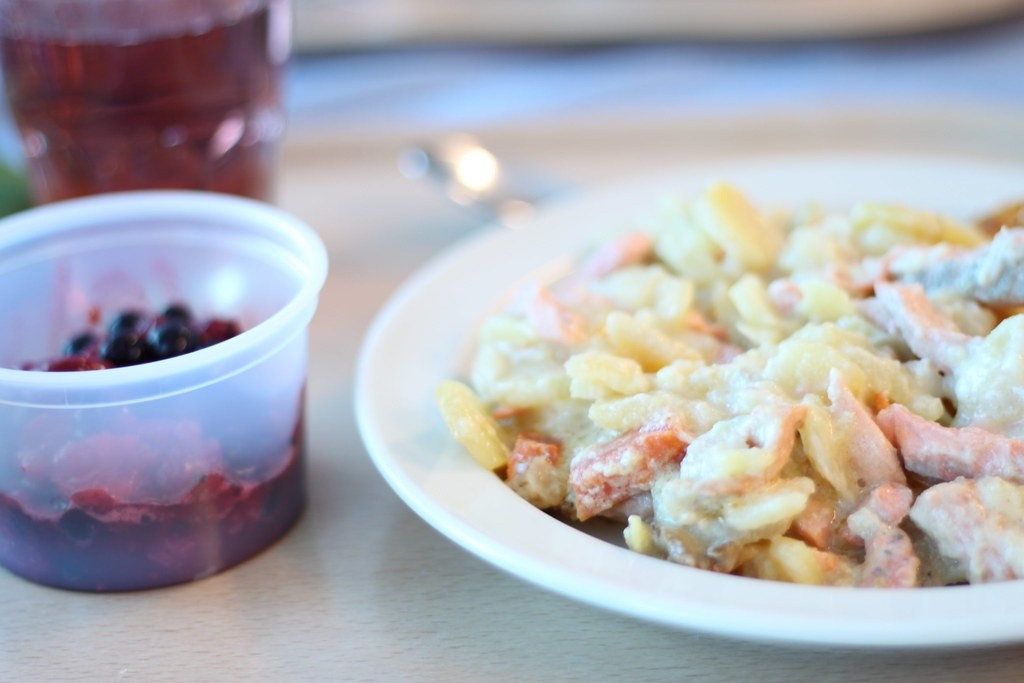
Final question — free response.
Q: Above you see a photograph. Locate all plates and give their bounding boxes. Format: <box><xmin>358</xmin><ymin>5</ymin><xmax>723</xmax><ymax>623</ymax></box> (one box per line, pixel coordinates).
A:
<box><xmin>354</xmin><ymin>158</ymin><xmax>1024</xmax><ymax>649</ymax></box>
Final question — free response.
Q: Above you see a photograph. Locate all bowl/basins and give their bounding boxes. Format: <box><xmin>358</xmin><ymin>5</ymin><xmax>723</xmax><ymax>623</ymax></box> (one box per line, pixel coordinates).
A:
<box><xmin>0</xmin><ymin>190</ymin><xmax>327</xmax><ymax>594</ymax></box>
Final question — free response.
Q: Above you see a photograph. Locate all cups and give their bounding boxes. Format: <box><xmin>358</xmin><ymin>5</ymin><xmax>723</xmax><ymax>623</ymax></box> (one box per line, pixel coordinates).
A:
<box><xmin>0</xmin><ymin>0</ymin><xmax>292</xmax><ymax>207</ymax></box>
